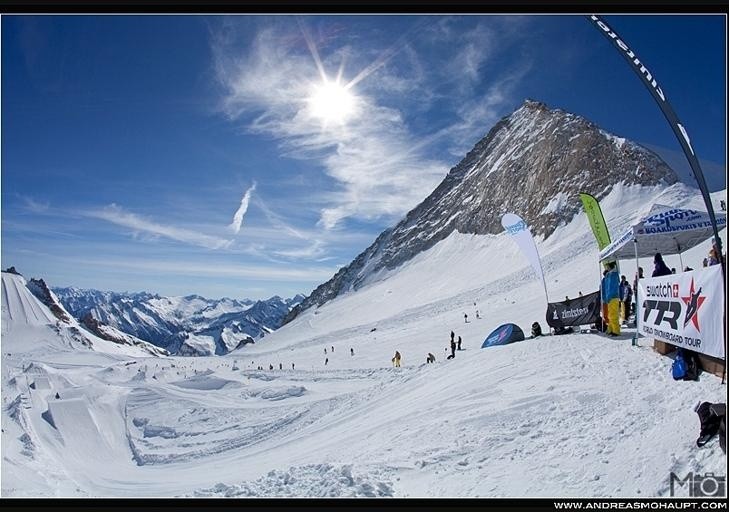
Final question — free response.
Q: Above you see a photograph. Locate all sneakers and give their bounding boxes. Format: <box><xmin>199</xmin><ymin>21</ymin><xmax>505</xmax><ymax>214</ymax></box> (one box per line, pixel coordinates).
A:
<box><xmin>696</xmin><ymin>401</ymin><xmax>720</xmax><ymax>446</ymax></box>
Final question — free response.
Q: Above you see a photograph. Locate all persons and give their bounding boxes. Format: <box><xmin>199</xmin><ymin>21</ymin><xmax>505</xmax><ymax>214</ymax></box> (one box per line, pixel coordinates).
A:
<box><xmin>563</xmin><ymin>237</ymin><xmax>723</xmax><ymax>338</ymax></box>
<box><xmin>257</xmin><ymin>302</ymin><xmax>479</xmax><ymax>369</ymax></box>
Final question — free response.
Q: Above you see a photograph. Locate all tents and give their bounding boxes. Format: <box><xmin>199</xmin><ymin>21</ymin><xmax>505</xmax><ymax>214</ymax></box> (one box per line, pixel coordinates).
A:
<box><xmin>481</xmin><ymin>323</ymin><xmax>524</xmax><ymax>348</ymax></box>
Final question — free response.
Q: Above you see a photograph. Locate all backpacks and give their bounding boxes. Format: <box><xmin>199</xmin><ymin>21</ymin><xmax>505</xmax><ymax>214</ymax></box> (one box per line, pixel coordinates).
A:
<box><xmin>672</xmin><ymin>349</ymin><xmax>702</xmax><ymax>380</ymax></box>
